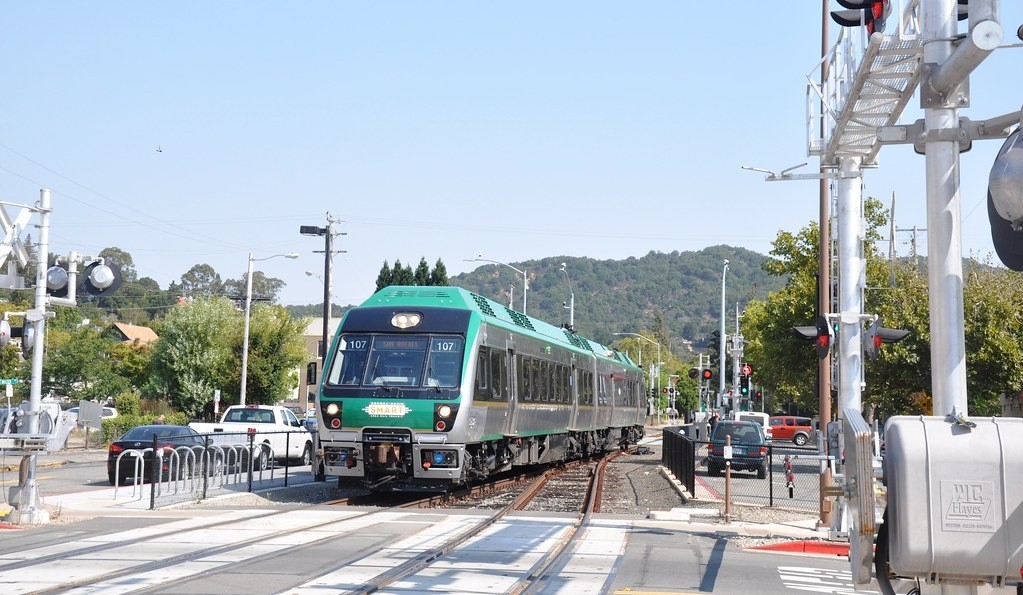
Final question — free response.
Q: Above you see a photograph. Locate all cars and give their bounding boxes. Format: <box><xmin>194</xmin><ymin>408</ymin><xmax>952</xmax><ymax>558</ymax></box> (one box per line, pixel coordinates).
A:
<box><xmin>108</xmin><ymin>425</ymin><xmax>222</xmax><ymax>487</ymax></box>
<box><xmin>283</xmin><ymin>405</ymin><xmax>318</xmax><ymax>432</ymax></box>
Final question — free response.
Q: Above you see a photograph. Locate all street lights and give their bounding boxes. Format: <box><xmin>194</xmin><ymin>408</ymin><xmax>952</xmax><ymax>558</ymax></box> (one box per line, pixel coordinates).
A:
<box><xmin>462</xmin><ymin>257</ymin><xmax>526</xmax><ymax>315</ymax></box>
<box><xmin>297</xmin><ymin>224</ymin><xmax>330</xmax><ymax>370</ymax></box>
<box><xmin>240</xmin><ymin>252</ymin><xmax>299</xmax><ymax>405</ymax></box>
<box><xmin>613</xmin><ymin>332</ymin><xmax>661</xmax><ymax>425</ymax></box>
<box><xmin>559</xmin><ymin>267</ymin><xmax>576</xmax><ymax>330</ymax></box>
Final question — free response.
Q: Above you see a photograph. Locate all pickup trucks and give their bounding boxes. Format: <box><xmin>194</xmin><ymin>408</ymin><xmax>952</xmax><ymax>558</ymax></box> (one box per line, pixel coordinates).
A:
<box><xmin>768</xmin><ymin>415</ymin><xmax>812</xmax><ymax>446</ymax></box>
<box><xmin>188</xmin><ymin>405</ymin><xmax>314</xmax><ymax>471</ymax></box>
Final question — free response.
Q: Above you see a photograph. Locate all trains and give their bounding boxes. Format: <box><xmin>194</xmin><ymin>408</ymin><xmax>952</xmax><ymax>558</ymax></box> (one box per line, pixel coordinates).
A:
<box><xmin>315</xmin><ymin>285</ymin><xmax>647</xmax><ymax>495</ymax></box>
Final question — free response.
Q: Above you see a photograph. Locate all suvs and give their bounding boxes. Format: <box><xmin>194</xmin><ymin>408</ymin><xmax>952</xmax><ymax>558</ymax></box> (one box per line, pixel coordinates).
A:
<box><xmin>707</xmin><ymin>419</ymin><xmax>772</xmax><ymax>478</ymax></box>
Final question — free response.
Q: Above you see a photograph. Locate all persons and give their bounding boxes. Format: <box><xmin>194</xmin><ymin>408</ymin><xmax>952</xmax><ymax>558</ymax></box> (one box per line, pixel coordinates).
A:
<box><xmin>709</xmin><ymin>413</ymin><xmax>722</xmax><ymax>440</ymax></box>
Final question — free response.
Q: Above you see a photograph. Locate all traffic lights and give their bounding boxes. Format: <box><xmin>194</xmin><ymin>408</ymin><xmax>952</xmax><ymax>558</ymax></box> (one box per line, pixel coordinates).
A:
<box><xmin>830</xmin><ymin>0</ymin><xmax>892</xmax><ymax>37</ymax></box>
<box><xmin>651</xmin><ymin>388</ymin><xmax>656</xmax><ymax>398</ymax></box>
<box><xmin>791</xmin><ymin>317</ymin><xmax>829</xmax><ymax>359</ymax></box>
<box><xmin>709</xmin><ymin>329</ymin><xmax>721</xmax><ymax>353</ymax></box>
<box><xmin>10</xmin><ymin>318</ymin><xmax>35</xmax><ymax>360</ymax></box>
<box><xmin>662</xmin><ymin>388</ymin><xmax>674</xmax><ymax>393</ymax></box>
<box><xmin>688</xmin><ymin>368</ymin><xmax>713</xmax><ymax>379</ymax></box>
<box><xmin>741</xmin><ymin>374</ymin><xmax>749</xmax><ymax>396</ymax></box>
<box><xmin>866</xmin><ymin>320</ymin><xmax>911</xmax><ymax>358</ymax></box>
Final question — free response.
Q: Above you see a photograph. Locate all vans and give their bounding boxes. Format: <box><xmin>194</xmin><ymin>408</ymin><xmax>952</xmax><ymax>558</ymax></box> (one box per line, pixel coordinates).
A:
<box><xmin>734</xmin><ymin>411</ymin><xmax>773</xmax><ymax>454</ymax></box>
